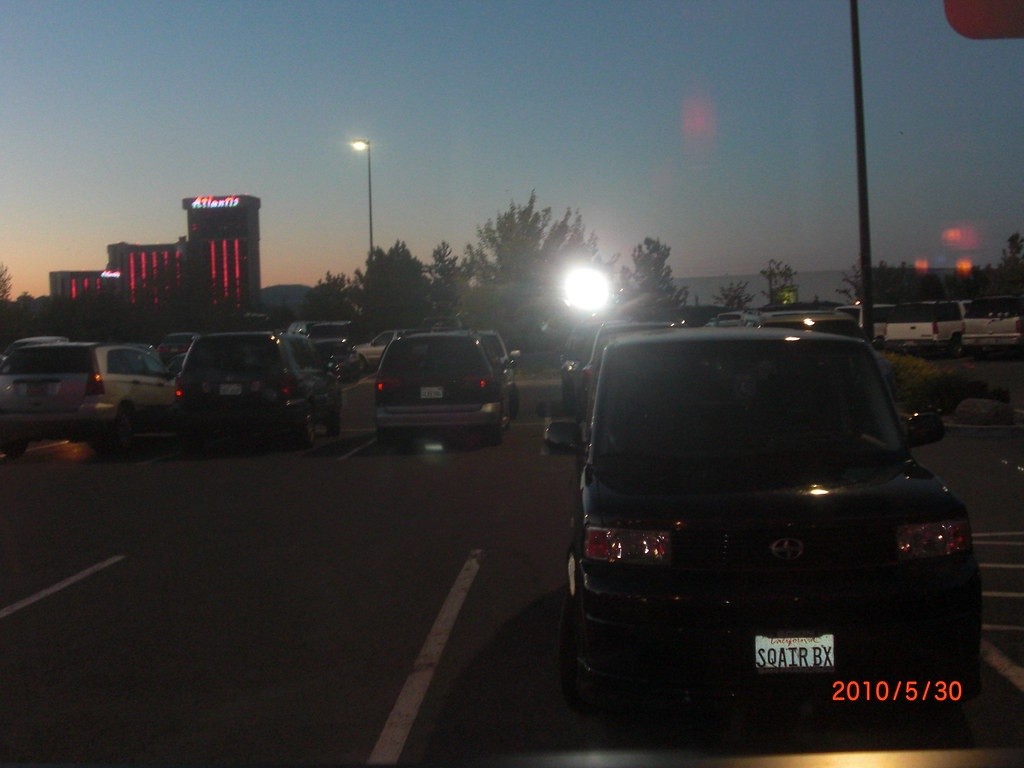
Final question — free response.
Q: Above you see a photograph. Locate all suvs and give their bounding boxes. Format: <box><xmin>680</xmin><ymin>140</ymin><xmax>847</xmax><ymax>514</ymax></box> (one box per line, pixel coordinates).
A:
<box><xmin>0</xmin><ymin>342</ymin><xmax>176</xmax><ymax>460</ymax></box>
<box><xmin>351</xmin><ymin>329</ymin><xmax>421</xmax><ymax>373</ymax></box>
<box><xmin>166</xmin><ymin>331</ymin><xmax>343</xmax><ymax>449</ymax></box>
<box><xmin>306</xmin><ymin>322</ymin><xmax>361</xmax><ymax>353</ymax></box>
<box><xmin>884</xmin><ymin>301</ymin><xmax>965</xmax><ymax>352</ymax></box>
<box><xmin>960</xmin><ymin>296</ymin><xmax>1024</xmax><ymax>349</ymax></box>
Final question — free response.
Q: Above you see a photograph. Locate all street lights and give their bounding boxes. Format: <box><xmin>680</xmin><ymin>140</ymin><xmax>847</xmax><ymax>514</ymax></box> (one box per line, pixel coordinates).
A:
<box><xmin>352</xmin><ymin>140</ymin><xmax>374</xmax><ymax>259</ymax></box>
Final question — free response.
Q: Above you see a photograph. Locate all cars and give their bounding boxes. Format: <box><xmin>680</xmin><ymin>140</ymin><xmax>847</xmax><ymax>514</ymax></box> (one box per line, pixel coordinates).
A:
<box><xmin>749</xmin><ymin>312</ymin><xmax>871</xmax><ymax>345</ymax></box>
<box><xmin>286</xmin><ymin>320</ymin><xmax>323</xmax><ymax>335</ymax></box>
<box><xmin>157</xmin><ymin>333</ymin><xmax>200</xmax><ymax>364</ymax></box>
<box><xmin>835</xmin><ymin>304</ymin><xmax>893</xmax><ymax>348</ymax></box>
<box><xmin>373</xmin><ymin>327</ymin><xmax>520</xmax><ymax>447</ymax></box>
<box><xmin>704</xmin><ymin>310</ymin><xmax>765</xmax><ymax>328</ymax></box>
<box><xmin>0</xmin><ymin>336</ymin><xmax>69</xmax><ymax>368</ymax></box>
<box><xmin>544</xmin><ymin>324</ymin><xmax>983</xmax><ymax>725</ymax></box>
